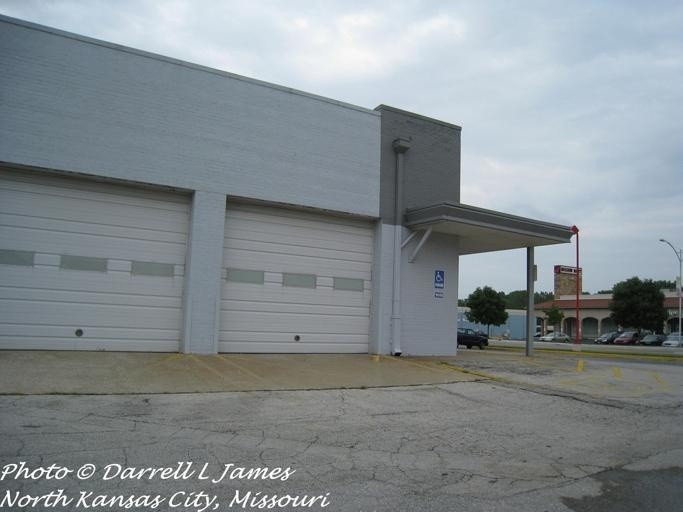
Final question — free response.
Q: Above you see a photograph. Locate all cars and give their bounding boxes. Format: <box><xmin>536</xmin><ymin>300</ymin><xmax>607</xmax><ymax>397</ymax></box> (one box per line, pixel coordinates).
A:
<box><xmin>457</xmin><ymin>327</ymin><xmax>488</xmax><ymax>350</ymax></box>
<box><xmin>533</xmin><ymin>331</ymin><xmax>569</xmax><ymax>343</ymax></box>
<box><xmin>595</xmin><ymin>331</ymin><xmax>683</xmax><ymax>347</ymax></box>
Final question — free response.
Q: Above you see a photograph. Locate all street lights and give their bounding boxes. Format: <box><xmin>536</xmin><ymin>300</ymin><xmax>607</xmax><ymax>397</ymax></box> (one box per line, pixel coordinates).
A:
<box><xmin>659</xmin><ymin>238</ymin><xmax>683</xmax><ymax>347</ymax></box>
<box><xmin>570</xmin><ymin>225</ymin><xmax>580</xmax><ymax>344</ymax></box>
